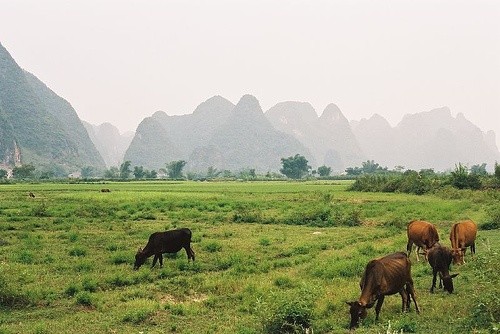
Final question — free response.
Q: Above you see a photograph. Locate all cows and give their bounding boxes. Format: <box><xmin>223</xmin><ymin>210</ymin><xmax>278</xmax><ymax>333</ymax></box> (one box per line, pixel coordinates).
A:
<box><xmin>405</xmin><ymin>219</ymin><xmax>440</xmax><ymax>262</ymax></box>
<box><xmin>345</xmin><ymin>250</ymin><xmax>420</xmax><ymax>329</ymax></box>
<box><xmin>449</xmin><ymin>218</ymin><xmax>478</xmax><ymax>266</ymax></box>
<box><xmin>133</xmin><ymin>227</ymin><xmax>196</xmax><ymax>272</ymax></box>
<box><xmin>426</xmin><ymin>241</ymin><xmax>459</xmax><ymax>295</ymax></box>
